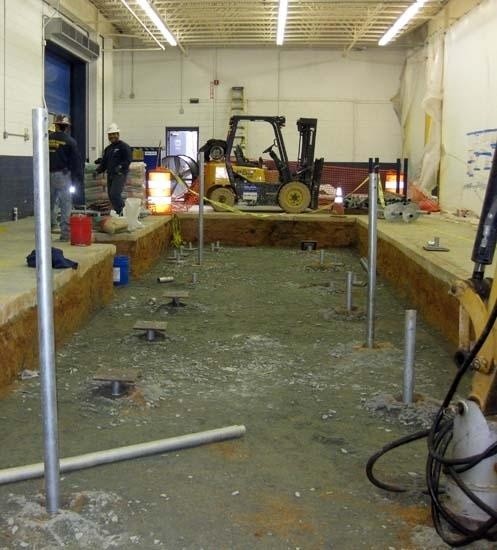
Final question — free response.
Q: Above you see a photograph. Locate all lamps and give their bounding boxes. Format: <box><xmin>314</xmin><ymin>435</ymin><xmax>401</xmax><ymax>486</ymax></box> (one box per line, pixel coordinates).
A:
<box><xmin>136</xmin><ymin>0</ymin><xmax>428</xmax><ymax>46</ymax></box>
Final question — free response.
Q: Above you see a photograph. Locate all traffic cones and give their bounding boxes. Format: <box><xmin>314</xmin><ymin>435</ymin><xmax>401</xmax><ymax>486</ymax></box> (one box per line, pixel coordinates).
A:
<box><xmin>331</xmin><ymin>182</ymin><xmax>349</xmax><ymax>217</ymax></box>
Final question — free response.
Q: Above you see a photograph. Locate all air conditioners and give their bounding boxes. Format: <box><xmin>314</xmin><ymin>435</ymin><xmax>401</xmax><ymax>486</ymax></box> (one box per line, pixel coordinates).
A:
<box><xmin>45</xmin><ymin>17</ymin><xmax>99</xmax><ymax>61</ymax></box>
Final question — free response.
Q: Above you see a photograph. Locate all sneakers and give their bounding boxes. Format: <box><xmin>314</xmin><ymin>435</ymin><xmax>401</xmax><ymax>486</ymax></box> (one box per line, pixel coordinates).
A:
<box><xmin>52</xmin><ymin>224</ymin><xmax>71</xmax><ymax>241</ymax></box>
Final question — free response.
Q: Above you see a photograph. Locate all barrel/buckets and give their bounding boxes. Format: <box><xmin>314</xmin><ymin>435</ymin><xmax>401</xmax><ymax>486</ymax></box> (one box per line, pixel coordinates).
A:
<box><xmin>70</xmin><ymin>215</ymin><xmax>92</xmax><ymax>244</ymax></box>
<box><xmin>113</xmin><ymin>255</ymin><xmax>129</xmax><ymax>285</ymax></box>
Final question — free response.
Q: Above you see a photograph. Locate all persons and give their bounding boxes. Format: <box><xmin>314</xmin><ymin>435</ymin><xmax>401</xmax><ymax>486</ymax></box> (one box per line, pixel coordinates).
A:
<box><xmin>92</xmin><ymin>122</ymin><xmax>132</xmax><ymax>215</ymax></box>
<box><xmin>49</xmin><ymin>113</ymin><xmax>82</xmax><ymax>241</ymax></box>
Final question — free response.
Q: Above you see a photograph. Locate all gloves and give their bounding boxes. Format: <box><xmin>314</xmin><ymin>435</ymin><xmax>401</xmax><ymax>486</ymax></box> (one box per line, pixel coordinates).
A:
<box><xmin>112</xmin><ymin>167</ymin><xmax>120</xmax><ymax>174</ymax></box>
<box><xmin>92</xmin><ymin>170</ymin><xmax>98</xmax><ymax>179</ymax></box>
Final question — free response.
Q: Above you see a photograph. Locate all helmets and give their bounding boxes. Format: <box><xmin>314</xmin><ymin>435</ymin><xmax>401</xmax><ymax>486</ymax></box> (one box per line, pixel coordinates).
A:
<box><xmin>107</xmin><ymin>124</ymin><xmax>120</xmax><ymax>133</ymax></box>
<box><xmin>52</xmin><ymin>114</ymin><xmax>70</xmax><ymax>125</ymax></box>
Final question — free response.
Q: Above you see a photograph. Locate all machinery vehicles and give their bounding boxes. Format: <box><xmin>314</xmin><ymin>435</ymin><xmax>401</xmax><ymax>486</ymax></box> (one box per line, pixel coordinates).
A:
<box><xmin>161</xmin><ymin>115</ymin><xmax>324</xmax><ymax>212</ymax></box>
